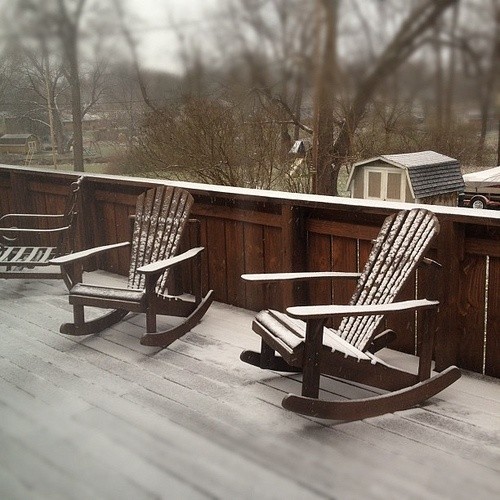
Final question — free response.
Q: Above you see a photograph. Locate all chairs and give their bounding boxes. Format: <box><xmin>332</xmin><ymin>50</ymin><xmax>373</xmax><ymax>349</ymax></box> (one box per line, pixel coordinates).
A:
<box><xmin>241</xmin><ymin>207</ymin><xmax>463</xmax><ymax>419</ymax></box>
<box><xmin>0</xmin><ymin>175</ymin><xmax>85</xmax><ymax>293</ymax></box>
<box><xmin>49</xmin><ymin>185</ymin><xmax>215</xmax><ymax>346</ymax></box>
